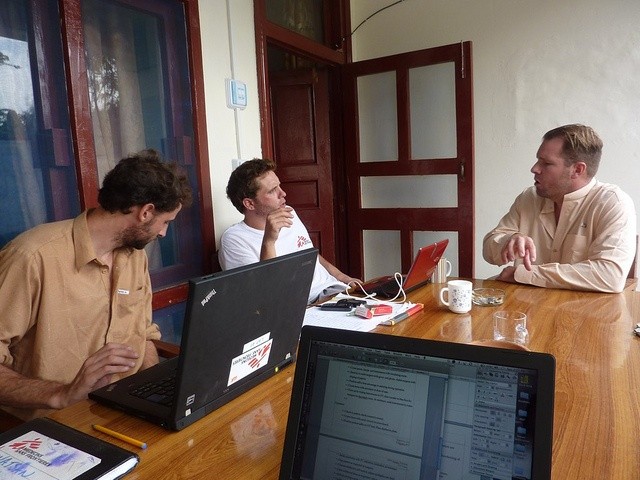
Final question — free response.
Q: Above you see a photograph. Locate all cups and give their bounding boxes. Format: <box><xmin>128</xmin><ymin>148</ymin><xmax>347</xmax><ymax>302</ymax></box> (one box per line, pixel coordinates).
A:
<box><xmin>430</xmin><ymin>257</ymin><xmax>451</xmax><ymax>283</ymax></box>
<box><xmin>439</xmin><ymin>279</ymin><xmax>472</xmax><ymax>315</ymax></box>
<box><xmin>492</xmin><ymin>312</ymin><xmax>529</xmax><ymax>348</ymax></box>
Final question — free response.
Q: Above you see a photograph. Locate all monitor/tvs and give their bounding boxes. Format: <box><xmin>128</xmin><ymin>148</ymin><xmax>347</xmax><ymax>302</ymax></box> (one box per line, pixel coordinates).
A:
<box><xmin>280</xmin><ymin>326</ymin><xmax>557</xmax><ymax>478</ymax></box>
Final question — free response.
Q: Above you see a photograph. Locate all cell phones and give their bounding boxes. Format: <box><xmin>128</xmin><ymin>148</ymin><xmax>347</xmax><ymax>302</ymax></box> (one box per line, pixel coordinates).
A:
<box><xmin>321</xmin><ymin>303</ymin><xmax>353</xmax><ymax>311</ymax></box>
<box><xmin>338</xmin><ymin>298</ymin><xmax>367</xmax><ymax>307</ymax></box>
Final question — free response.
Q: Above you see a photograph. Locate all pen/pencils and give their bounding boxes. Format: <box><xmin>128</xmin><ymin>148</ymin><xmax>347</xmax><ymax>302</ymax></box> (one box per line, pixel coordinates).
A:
<box><xmin>90</xmin><ymin>424</ymin><xmax>147</xmax><ymax>449</ymax></box>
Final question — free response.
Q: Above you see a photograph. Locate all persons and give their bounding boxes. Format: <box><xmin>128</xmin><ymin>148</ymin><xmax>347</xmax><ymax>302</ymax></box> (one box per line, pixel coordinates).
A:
<box><xmin>218</xmin><ymin>159</ymin><xmax>363</xmax><ymax>306</ymax></box>
<box><xmin>0</xmin><ymin>148</ymin><xmax>194</xmax><ymax>423</ymax></box>
<box><xmin>483</xmin><ymin>124</ymin><xmax>636</xmax><ymax>294</ymax></box>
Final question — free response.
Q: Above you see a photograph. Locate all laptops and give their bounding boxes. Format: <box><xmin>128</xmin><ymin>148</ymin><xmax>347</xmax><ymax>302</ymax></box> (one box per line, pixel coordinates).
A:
<box><xmin>88</xmin><ymin>245</ymin><xmax>318</xmax><ymax>431</ymax></box>
<box><xmin>355</xmin><ymin>238</ymin><xmax>450</xmax><ymax>298</ymax></box>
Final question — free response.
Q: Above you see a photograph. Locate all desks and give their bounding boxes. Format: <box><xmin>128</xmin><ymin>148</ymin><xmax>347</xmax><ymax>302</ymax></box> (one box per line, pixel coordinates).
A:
<box><xmin>1</xmin><ymin>277</ymin><xmax>639</xmax><ymax>476</ymax></box>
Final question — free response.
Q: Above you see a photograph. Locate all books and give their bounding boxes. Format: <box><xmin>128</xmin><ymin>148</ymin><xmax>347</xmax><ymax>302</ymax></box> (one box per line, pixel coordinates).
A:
<box><xmin>0</xmin><ymin>417</ymin><xmax>141</xmax><ymax>480</ymax></box>
<box><xmin>378</xmin><ymin>302</ymin><xmax>424</xmax><ymax>325</ymax></box>
